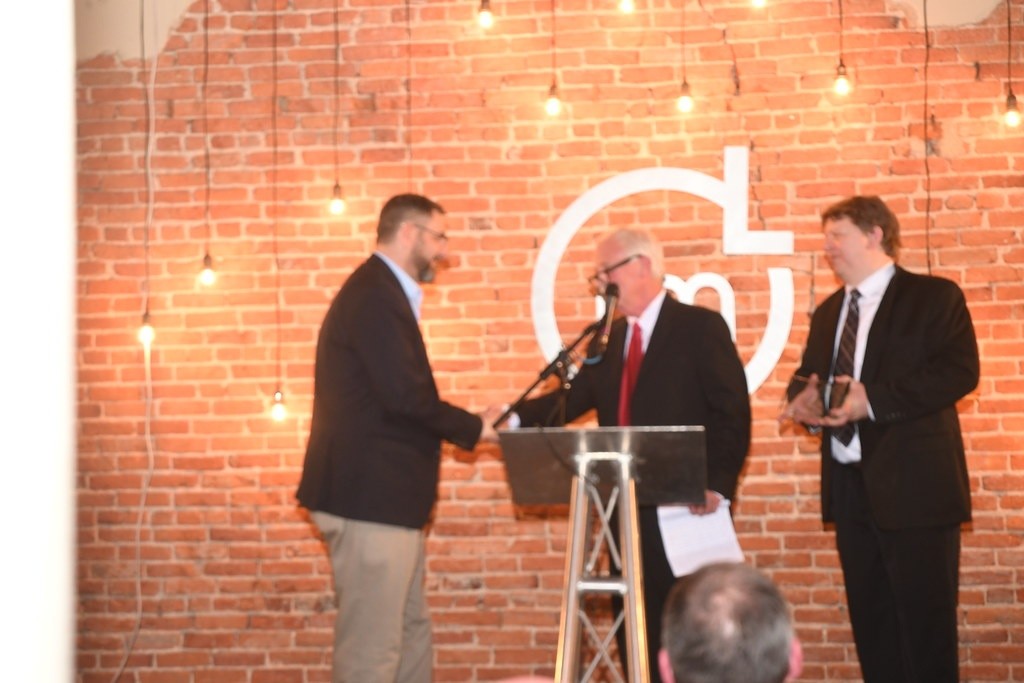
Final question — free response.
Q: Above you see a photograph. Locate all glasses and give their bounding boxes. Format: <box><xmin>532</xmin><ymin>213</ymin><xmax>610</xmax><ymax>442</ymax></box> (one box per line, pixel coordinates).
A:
<box><xmin>413</xmin><ymin>223</ymin><xmax>450</xmax><ymax>250</ymax></box>
<box><xmin>587</xmin><ymin>253</ymin><xmax>643</xmax><ymax>288</ymax></box>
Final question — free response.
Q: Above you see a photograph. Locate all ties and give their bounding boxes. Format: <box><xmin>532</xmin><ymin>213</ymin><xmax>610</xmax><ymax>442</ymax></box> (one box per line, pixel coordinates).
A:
<box><xmin>616</xmin><ymin>322</ymin><xmax>645</xmax><ymax>426</ymax></box>
<box><xmin>828</xmin><ymin>287</ymin><xmax>863</xmax><ymax>448</ymax></box>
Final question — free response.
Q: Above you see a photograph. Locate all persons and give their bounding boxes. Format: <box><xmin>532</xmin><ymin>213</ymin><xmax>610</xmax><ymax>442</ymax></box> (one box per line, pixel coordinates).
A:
<box><xmin>782</xmin><ymin>195</ymin><xmax>981</xmax><ymax>683</ymax></box>
<box><xmin>658</xmin><ymin>563</ymin><xmax>804</xmax><ymax>683</ymax></box>
<box><xmin>294</xmin><ymin>191</ymin><xmax>507</xmax><ymax>683</ymax></box>
<box><xmin>485</xmin><ymin>224</ymin><xmax>752</xmax><ymax>683</ymax></box>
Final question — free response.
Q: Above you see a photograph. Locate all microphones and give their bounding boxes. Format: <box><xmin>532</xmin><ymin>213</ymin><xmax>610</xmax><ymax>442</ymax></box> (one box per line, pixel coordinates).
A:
<box><xmin>596</xmin><ymin>284</ymin><xmax>619</xmax><ymax>354</ymax></box>
